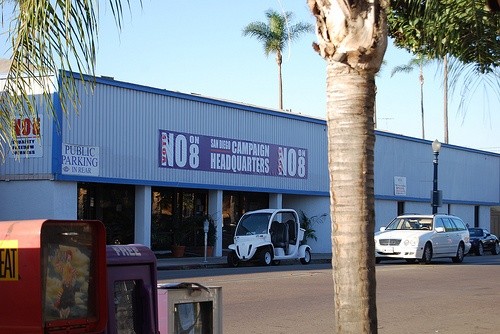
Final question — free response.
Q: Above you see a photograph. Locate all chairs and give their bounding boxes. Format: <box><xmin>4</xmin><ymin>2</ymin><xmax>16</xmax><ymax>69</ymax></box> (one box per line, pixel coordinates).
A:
<box><xmin>285</xmin><ymin>220</ymin><xmax>296</xmax><ymax>245</ymax></box>
<box><xmin>271</xmin><ymin>221</ymin><xmax>285</xmax><ymax>247</ymax></box>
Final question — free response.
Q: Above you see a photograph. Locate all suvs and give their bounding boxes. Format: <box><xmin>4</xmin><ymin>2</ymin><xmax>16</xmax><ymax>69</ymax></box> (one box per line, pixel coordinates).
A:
<box><xmin>374</xmin><ymin>212</ymin><xmax>472</xmax><ymax>265</ymax></box>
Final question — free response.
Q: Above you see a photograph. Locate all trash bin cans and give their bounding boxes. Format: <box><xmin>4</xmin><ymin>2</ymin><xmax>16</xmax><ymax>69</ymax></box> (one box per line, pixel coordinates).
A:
<box><xmin>158</xmin><ymin>281</ymin><xmax>223</xmax><ymax>334</ymax></box>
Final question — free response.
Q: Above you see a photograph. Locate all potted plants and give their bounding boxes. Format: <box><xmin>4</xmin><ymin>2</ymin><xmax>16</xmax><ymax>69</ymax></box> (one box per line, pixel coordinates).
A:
<box><xmin>197</xmin><ymin>215</ymin><xmax>216</xmax><ymax>257</ymax></box>
<box><xmin>161</xmin><ymin>191</ymin><xmax>193</xmax><ymax>257</ymax></box>
<box><xmin>299</xmin><ymin>208</ymin><xmax>328</xmax><ymax>263</ymax></box>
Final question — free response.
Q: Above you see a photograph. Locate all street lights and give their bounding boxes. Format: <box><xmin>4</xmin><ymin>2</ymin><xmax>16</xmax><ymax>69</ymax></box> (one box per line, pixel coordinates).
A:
<box><xmin>430</xmin><ymin>139</ymin><xmax>442</xmax><ymax>216</ymax></box>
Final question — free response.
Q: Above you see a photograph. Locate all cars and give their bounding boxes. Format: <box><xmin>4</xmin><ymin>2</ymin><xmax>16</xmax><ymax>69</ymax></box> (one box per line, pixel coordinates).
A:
<box><xmin>463</xmin><ymin>227</ymin><xmax>500</xmax><ymax>256</ymax></box>
